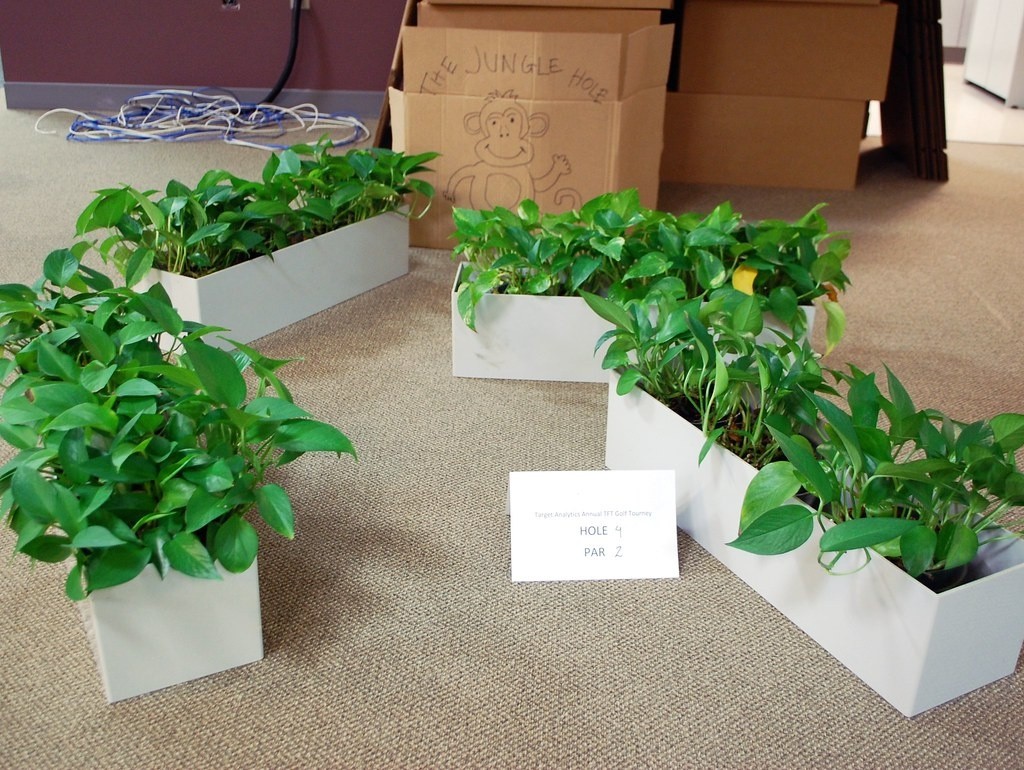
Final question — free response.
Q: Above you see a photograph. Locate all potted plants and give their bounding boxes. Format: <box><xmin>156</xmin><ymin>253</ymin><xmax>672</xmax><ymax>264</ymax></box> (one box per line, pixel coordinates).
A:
<box><xmin>450</xmin><ymin>189</ymin><xmax>852</xmax><ymax>383</ymax></box>
<box><xmin>576</xmin><ymin>274</ymin><xmax>1024</xmax><ymax>592</ymax></box>
<box><xmin>74</xmin><ymin>131</ymin><xmax>440</xmax><ymax>356</ymax></box>
<box><xmin>1</xmin><ymin>237</ymin><xmax>359</xmax><ymax>703</ymax></box>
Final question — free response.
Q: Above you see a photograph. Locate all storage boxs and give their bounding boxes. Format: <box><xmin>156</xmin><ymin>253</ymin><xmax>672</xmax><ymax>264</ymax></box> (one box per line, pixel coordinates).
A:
<box><xmin>387</xmin><ymin>0</ymin><xmax>676</xmax><ymax>252</ymax></box>
<box><xmin>677</xmin><ymin>2</ymin><xmax>898</xmax><ymax>103</ymax></box>
<box><xmin>661</xmin><ymin>88</ymin><xmax>870</xmax><ymax>196</ymax></box>
<box><xmin>602</xmin><ymin>363</ymin><xmax>1024</xmax><ymax>717</ymax></box>
